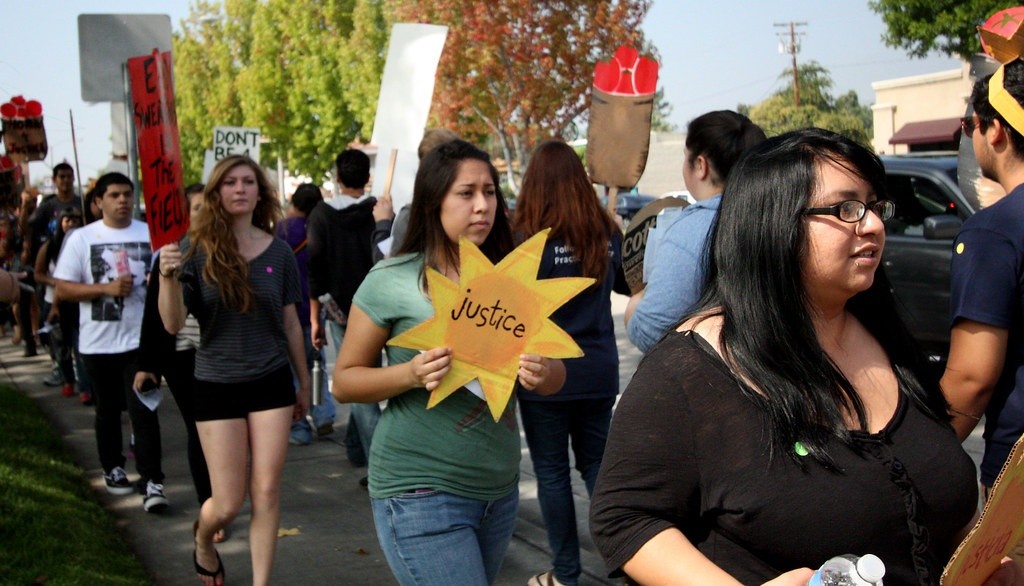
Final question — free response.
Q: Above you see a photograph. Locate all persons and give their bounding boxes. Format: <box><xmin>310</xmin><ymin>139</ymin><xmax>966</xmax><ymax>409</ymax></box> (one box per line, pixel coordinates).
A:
<box><xmin>52</xmin><ymin>171</ymin><xmax>169</xmax><ymax>512</ymax></box>
<box><xmin>331</xmin><ymin>138</ymin><xmax>566</xmax><ymax>586</ymax></box>
<box><xmin>0</xmin><ymin>154</ymin><xmax>103</xmax><ymax>405</ymax></box>
<box><xmin>589</xmin><ymin>129</ymin><xmax>1024</xmax><ymax>586</ymax></box>
<box><xmin>306</xmin><ymin>149</ymin><xmax>396</xmax><ymax>486</ymax></box>
<box><xmin>132</xmin><ymin>183</ymin><xmax>229</xmax><ymax>543</ymax></box>
<box><xmin>624</xmin><ymin>110</ymin><xmax>768</xmax><ymax>353</ymax></box>
<box><xmin>273</xmin><ymin>182</ymin><xmax>334</xmax><ymax>446</ymax></box>
<box><xmin>157</xmin><ymin>154</ymin><xmax>311</xmax><ymax>586</ymax></box>
<box><xmin>509</xmin><ymin>141</ymin><xmax>633</xmax><ymax>586</ymax></box>
<box><xmin>938</xmin><ymin>58</ymin><xmax>1024</xmax><ymax>572</ymax></box>
<box><xmin>372</xmin><ymin>129</ymin><xmax>461</xmax><ymax>266</ymax></box>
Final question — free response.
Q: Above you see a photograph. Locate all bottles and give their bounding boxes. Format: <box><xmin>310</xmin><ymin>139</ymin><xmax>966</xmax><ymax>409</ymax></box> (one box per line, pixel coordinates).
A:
<box><xmin>114</xmin><ymin>249</ymin><xmax>131</xmax><ymax>282</ymax></box>
<box><xmin>807</xmin><ymin>554</ymin><xmax>886</xmax><ymax>586</ymax></box>
<box><xmin>312</xmin><ymin>351</ymin><xmax>323</xmax><ymax>406</ymax></box>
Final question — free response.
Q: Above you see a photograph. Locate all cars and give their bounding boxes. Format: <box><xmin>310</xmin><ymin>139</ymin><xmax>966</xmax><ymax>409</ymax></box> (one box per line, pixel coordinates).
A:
<box><xmin>598</xmin><ymin>190</ymin><xmax>697</xmax><ymax>222</ymax></box>
<box><xmin>876</xmin><ymin>151</ymin><xmax>976</xmax><ymax>344</ymax></box>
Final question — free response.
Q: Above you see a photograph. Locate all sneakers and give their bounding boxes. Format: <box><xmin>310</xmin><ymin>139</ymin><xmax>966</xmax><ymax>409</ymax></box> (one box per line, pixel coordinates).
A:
<box><xmin>141</xmin><ymin>479</ymin><xmax>170</xmax><ymax>512</ymax></box>
<box><xmin>101</xmin><ymin>464</ymin><xmax>134</xmax><ymax>496</ymax></box>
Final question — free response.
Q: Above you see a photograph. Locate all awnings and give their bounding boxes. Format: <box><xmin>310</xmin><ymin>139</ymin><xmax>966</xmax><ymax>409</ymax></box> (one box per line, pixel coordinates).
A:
<box><xmin>888</xmin><ymin>117</ymin><xmax>964</xmax><ymax>144</ymax></box>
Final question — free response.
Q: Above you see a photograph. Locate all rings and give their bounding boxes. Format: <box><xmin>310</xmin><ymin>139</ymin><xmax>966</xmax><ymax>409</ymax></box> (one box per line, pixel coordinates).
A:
<box><xmin>173</xmin><ymin>264</ymin><xmax>176</xmax><ymax>268</ymax></box>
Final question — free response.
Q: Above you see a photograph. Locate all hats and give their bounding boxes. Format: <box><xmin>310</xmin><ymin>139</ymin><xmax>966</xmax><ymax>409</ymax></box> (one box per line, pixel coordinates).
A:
<box><xmin>59</xmin><ymin>205</ymin><xmax>80</xmax><ymax>220</ymax></box>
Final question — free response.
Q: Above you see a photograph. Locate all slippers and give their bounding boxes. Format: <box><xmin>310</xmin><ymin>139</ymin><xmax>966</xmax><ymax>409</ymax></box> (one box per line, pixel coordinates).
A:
<box><xmin>192</xmin><ymin>519</ymin><xmax>226</xmax><ymax>586</ymax></box>
<box><xmin>528</xmin><ymin>568</ymin><xmax>556</xmax><ymax>586</ymax></box>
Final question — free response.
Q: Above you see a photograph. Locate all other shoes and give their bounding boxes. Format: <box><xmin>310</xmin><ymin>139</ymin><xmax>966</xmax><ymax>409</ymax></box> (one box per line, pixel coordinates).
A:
<box><xmin>315</xmin><ymin>423</ymin><xmax>335</xmax><ymax>436</ymax></box>
<box><xmin>62</xmin><ymin>383</ymin><xmax>74</xmax><ymax>399</ymax></box>
<box><xmin>79</xmin><ymin>390</ymin><xmax>93</xmax><ymax>404</ymax></box>
<box><xmin>43</xmin><ymin>368</ymin><xmax>65</xmax><ymax>386</ymax></box>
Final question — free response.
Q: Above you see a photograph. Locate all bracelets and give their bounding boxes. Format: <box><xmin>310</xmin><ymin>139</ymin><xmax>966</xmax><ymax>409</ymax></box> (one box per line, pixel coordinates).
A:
<box><xmin>159</xmin><ymin>271</ymin><xmax>173</xmax><ymax>277</ymax></box>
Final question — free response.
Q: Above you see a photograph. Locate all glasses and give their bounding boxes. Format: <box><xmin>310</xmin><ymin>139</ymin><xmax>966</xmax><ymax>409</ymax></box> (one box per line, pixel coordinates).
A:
<box><xmin>958</xmin><ymin>117</ymin><xmax>987</xmax><ymax>138</ymax></box>
<box><xmin>798</xmin><ymin>199</ymin><xmax>895</xmax><ymax>225</ymax></box>
